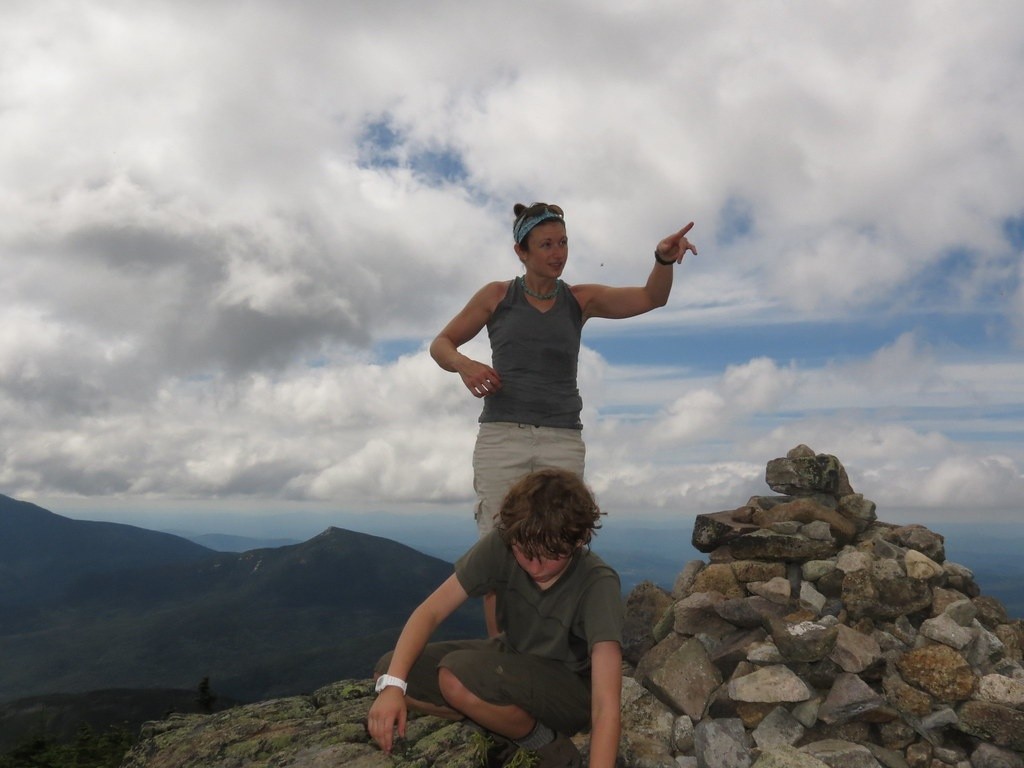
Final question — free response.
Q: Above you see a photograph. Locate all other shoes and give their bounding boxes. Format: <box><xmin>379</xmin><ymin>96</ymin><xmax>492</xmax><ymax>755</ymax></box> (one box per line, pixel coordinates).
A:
<box><xmin>529</xmin><ymin>731</ymin><xmax>582</xmax><ymax>768</ymax></box>
<box><xmin>482</xmin><ymin>731</ymin><xmax>523</xmax><ymax>768</ymax></box>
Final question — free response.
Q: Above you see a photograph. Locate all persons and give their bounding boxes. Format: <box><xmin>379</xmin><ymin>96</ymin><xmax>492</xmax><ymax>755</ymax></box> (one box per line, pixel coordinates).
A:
<box><xmin>367</xmin><ymin>469</ymin><xmax>625</xmax><ymax>767</ymax></box>
<box><xmin>430</xmin><ymin>201</ymin><xmax>698</xmax><ymax>639</ymax></box>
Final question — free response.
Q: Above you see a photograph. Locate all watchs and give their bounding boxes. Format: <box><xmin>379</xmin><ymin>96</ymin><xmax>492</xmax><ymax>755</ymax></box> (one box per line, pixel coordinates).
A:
<box><xmin>374</xmin><ymin>674</ymin><xmax>409</xmax><ymax>695</ymax></box>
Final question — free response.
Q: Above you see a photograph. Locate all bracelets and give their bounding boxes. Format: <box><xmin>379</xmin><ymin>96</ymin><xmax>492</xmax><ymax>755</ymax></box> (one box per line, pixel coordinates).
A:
<box><xmin>654</xmin><ymin>251</ymin><xmax>677</xmax><ymax>265</ymax></box>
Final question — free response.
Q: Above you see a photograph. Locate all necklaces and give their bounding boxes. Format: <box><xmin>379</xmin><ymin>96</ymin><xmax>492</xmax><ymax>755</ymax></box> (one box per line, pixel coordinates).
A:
<box><xmin>520</xmin><ymin>274</ymin><xmax>561</xmax><ymax>300</ymax></box>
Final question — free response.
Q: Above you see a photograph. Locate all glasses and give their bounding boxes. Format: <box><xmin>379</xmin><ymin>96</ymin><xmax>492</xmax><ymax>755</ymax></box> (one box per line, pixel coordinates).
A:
<box><xmin>516</xmin><ymin>204</ymin><xmax>564</xmax><ymax>244</ymax></box>
